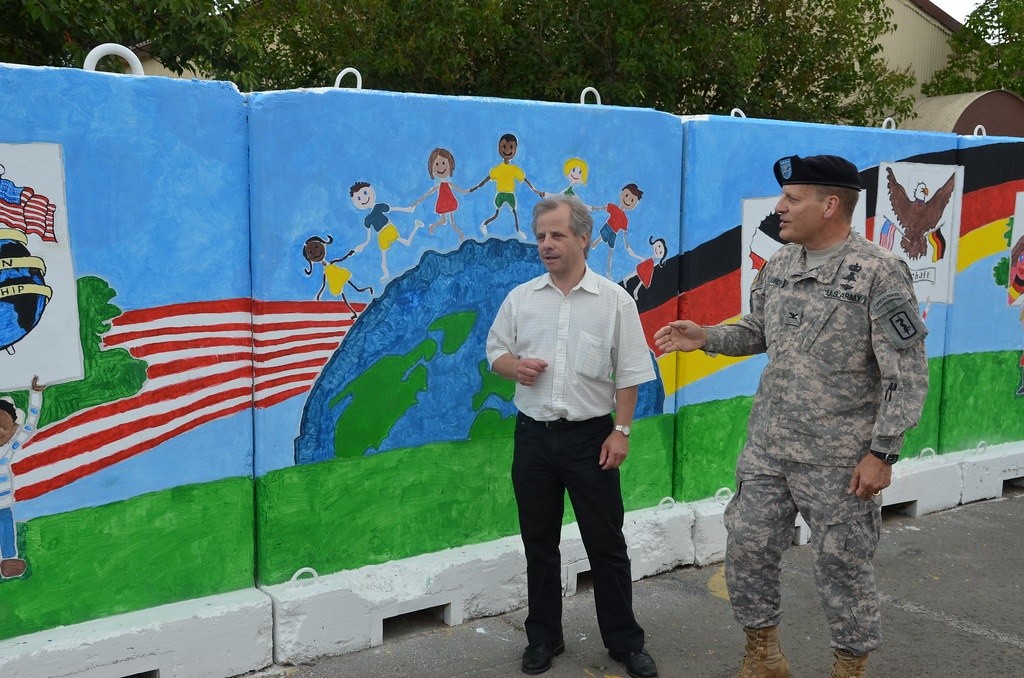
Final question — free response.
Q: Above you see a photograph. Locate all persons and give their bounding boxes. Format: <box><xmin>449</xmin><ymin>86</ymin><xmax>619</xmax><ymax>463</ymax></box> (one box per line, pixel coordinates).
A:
<box><xmin>653</xmin><ymin>154</ymin><xmax>929</xmax><ymax>678</ymax></box>
<box><xmin>485</xmin><ymin>194</ymin><xmax>658</xmax><ymax>678</ymax></box>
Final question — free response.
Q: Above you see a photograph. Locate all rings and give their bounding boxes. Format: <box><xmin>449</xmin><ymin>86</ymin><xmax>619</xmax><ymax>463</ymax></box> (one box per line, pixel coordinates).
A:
<box><xmin>872</xmin><ymin>490</ymin><xmax>881</xmax><ymax>496</ymax></box>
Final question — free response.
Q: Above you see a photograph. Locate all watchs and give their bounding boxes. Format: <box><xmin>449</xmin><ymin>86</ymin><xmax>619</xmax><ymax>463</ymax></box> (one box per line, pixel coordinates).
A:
<box><xmin>869</xmin><ymin>448</ymin><xmax>900</xmax><ymax>467</ymax></box>
<box><xmin>613</xmin><ymin>424</ymin><xmax>632</xmax><ymax>439</ymax></box>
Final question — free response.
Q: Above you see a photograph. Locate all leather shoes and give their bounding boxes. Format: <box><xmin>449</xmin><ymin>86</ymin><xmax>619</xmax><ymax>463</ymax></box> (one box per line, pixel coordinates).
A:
<box><xmin>521</xmin><ymin>639</ymin><xmax>565</xmax><ymax>675</ymax></box>
<box><xmin>610</xmin><ymin>645</ymin><xmax>658</xmax><ymax>678</ymax></box>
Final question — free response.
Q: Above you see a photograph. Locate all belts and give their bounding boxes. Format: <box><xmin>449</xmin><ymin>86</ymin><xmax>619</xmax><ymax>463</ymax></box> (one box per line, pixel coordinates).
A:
<box><xmin>518</xmin><ymin>411</ymin><xmax>611</xmax><ymax>431</ymax></box>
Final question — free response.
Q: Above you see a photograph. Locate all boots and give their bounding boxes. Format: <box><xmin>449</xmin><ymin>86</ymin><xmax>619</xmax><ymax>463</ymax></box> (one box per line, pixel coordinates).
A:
<box><xmin>727</xmin><ymin>626</ymin><xmax>792</xmax><ymax>678</ymax></box>
<box><xmin>829</xmin><ymin>650</ymin><xmax>868</xmax><ymax>678</ymax></box>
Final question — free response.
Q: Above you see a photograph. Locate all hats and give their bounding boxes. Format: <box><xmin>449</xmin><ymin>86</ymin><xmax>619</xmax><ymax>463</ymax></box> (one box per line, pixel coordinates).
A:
<box><xmin>773</xmin><ymin>154</ymin><xmax>862</xmax><ymax>193</ymax></box>
